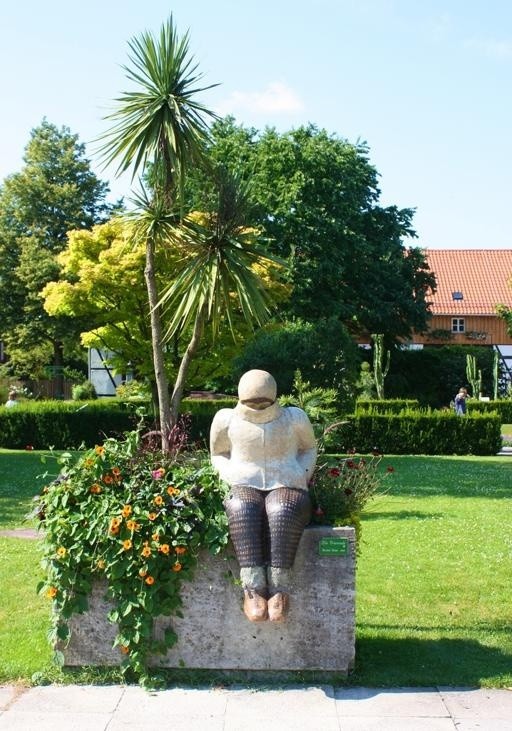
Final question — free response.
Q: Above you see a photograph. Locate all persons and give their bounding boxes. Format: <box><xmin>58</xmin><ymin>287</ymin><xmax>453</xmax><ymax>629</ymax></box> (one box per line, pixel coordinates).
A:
<box><xmin>210</xmin><ymin>370</ymin><xmax>317</xmax><ymax>622</ymax></box>
<box><xmin>5</xmin><ymin>391</ymin><xmax>18</xmax><ymax>408</ymax></box>
<box><xmin>455</xmin><ymin>387</ymin><xmax>467</xmax><ymax>415</ymax></box>
